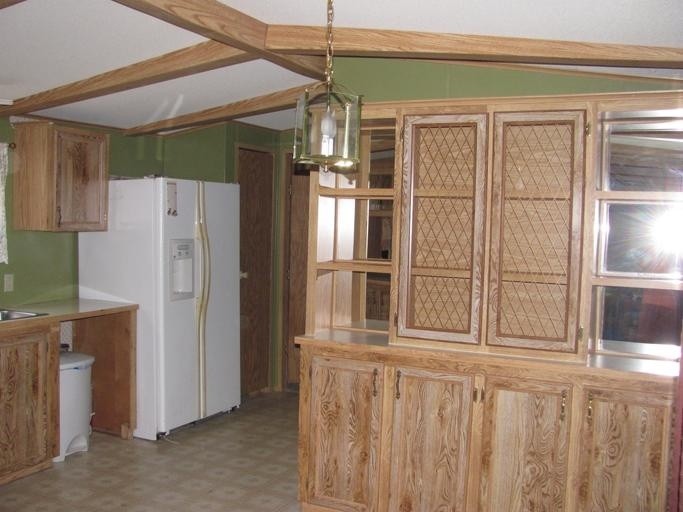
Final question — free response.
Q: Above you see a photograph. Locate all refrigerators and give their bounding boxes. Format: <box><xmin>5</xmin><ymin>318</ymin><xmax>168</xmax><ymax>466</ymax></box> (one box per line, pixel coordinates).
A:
<box><xmin>79</xmin><ymin>177</ymin><xmax>241</xmax><ymax>441</ymax></box>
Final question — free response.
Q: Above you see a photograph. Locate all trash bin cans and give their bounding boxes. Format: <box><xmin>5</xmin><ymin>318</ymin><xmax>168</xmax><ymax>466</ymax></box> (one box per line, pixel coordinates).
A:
<box><xmin>53</xmin><ymin>353</ymin><xmax>96</xmax><ymax>457</ymax></box>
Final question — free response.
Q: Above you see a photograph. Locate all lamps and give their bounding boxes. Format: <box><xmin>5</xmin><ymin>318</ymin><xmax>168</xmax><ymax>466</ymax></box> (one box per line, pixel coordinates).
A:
<box><xmin>290</xmin><ymin>0</ymin><xmax>363</xmax><ymax>175</ymax></box>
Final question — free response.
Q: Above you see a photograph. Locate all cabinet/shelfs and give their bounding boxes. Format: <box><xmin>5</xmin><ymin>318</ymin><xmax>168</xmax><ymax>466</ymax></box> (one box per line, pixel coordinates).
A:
<box><xmin>0</xmin><ymin>316</ymin><xmax>59</xmax><ymax>486</ymax></box>
<box><xmin>398</xmin><ymin>106</ymin><xmax>593</xmax><ymax>366</ymax></box>
<box><xmin>12</xmin><ymin>120</ymin><xmax>110</xmax><ymax>233</ymax></box>
<box><xmin>588</xmin><ymin>97</ymin><xmax>681</xmax><ymax>375</ymax></box>
<box><xmin>474</xmin><ymin>359</ymin><xmax>681</xmax><ymax>510</ymax></box>
<box><xmin>297</xmin><ymin>98</ymin><xmax>403</xmax><ymax>345</ymax></box>
<box><xmin>300</xmin><ymin>346</ymin><xmax>474</xmax><ymax>510</ymax></box>
